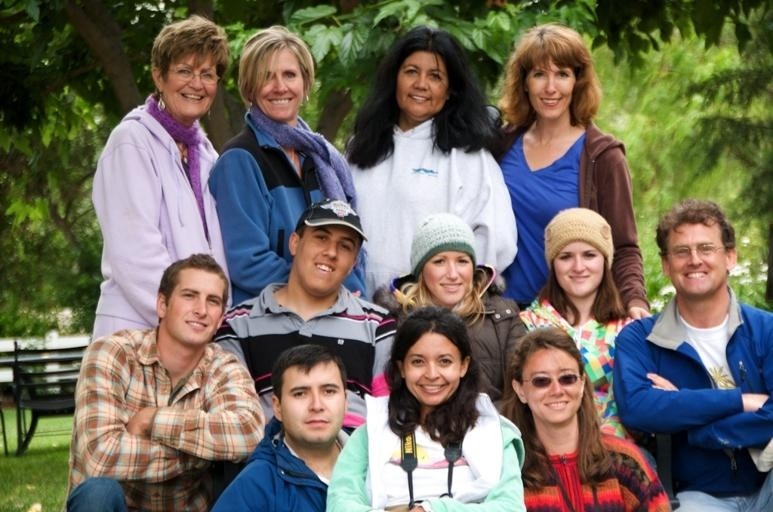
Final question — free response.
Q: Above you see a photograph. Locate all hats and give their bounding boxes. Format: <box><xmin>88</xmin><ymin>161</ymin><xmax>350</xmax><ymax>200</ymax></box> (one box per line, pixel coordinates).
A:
<box><xmin>295</xmin><ymin>198</ymin><xmax>369</xmax><ymax>242</ymax></box>
<box><xmin>411</xmin><ymin>212</ymin><xmax>477</xmax><ymax>277</ymax></box>
<box><xmin>544</xmin><ymin>207</ymin><xmax>614</xmax><ymax>272</ymax></box>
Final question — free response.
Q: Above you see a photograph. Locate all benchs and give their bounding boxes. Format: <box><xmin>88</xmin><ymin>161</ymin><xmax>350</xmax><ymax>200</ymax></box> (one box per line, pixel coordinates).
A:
<box><xmin>0</xmin><ymin>341</ymin><xmax>88</xmax><ymax>456</ymax></box>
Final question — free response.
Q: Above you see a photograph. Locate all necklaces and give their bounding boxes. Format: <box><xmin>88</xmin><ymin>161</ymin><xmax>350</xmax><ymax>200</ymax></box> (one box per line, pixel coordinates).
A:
<box><xmin>179</xmin><ymin>145</ymin><xmax>191</xmax><ymax>168</ymax></box>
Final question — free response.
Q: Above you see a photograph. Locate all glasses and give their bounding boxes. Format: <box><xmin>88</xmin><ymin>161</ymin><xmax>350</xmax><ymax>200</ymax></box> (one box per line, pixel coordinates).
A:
<box><xmin>168</xmin><ymin>67</ymin><xmax>221</xmax><ymax>85</ymax></box>
<box><xmin>667</xmin><ymin>244</ymin><xmax>725</xmax><ymax>258</ymax></box>
<box><xmin>524</xmin><ymin>375</ymin><xmax>581</xmax><ymax>387</ymax></box>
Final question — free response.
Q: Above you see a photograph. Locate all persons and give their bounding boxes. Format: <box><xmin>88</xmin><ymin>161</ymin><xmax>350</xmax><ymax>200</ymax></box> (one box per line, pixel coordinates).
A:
<box><xmin>506</xmin><ymin>328</ymin><xmax>672</xmax><ymax>512</ymax></box>
<box><xmin>92</xmin><ymin>15</ymin><xmax>234</xmax><ymax>339</ymax></box>
<box><xmin>66</xmin><ymin>255</ymin><xmax>265</xmax><ymax>511</ymax></box>
<box><xmin>328</xmin><ymin>309</ymin><xmax>526</xmax><ymax>510</ymax></box>
<box><xmin>500</xmin><ymin>24</ymin><xmax>651</xmax><ymax>321</ymax></box>
<box><xmin>340</xmin><ymin>25</ymin><xmax>518</xmax><ymax>298</ymax></box>
<box><xmin>508</xmin><ymin>207</ymin><xmax>657</xmax><ymax>472</ymax></box>
<box><xmin>382</xmin><ymin>211</ymin><xmax>526</xmax><ymax>418</ymax></box>
<box><xmin>216</xmin><ymin>199</ymin><xmax>396</xmax><ymax>435</ymax></box>
<box><xmin>614</xmin><ymin>200</ymin><xmax>772</xmax><ymax>512</ymax></box>
<box><xmin>208</xmin><ymin>24</ymin><xmax>367</xmax><ymax>304</ymax></box>
<box><xmin>209</xmin><ymin>344</ymin><xmax>350</xmax><ymax>510</ymax></box>
